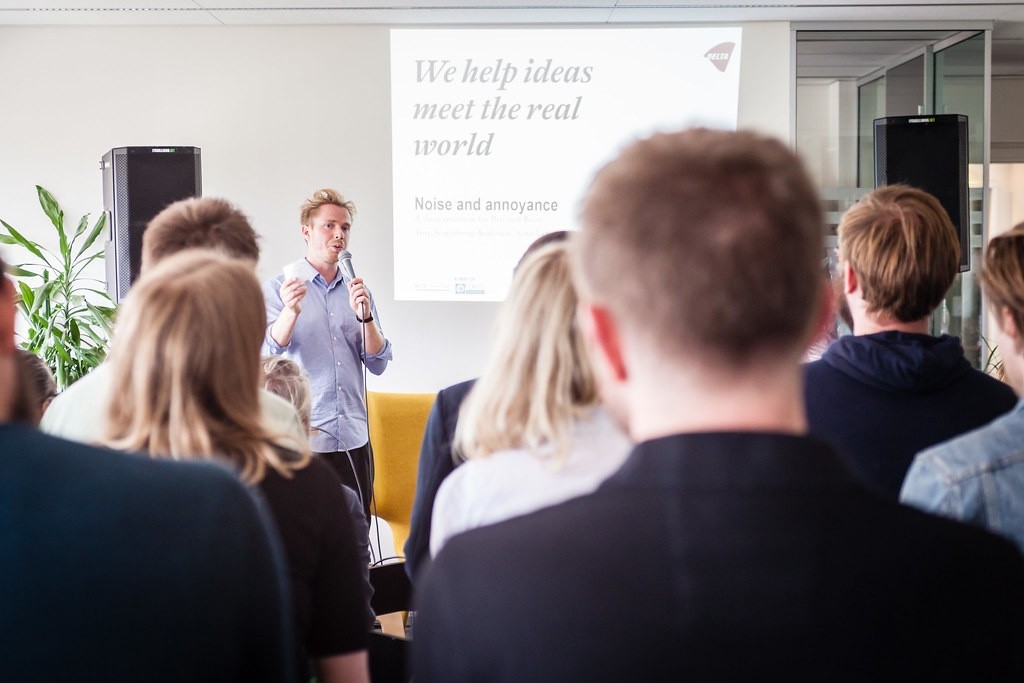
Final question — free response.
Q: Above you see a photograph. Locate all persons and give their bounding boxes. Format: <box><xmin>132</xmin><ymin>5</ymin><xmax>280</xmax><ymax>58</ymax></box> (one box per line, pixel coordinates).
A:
<box><xmin>256</xmin><ymin>189</ymin><xmax>390</xmax><ymax>530</ymax></box>
<box><xmin>400</xmin><ymin>130</ymin><xmax>1024</xmax><ymax>683</ymax></box>
<box><xmin>0</xmin><ymin>201</ymin><xmax>375</xmax><ymax>683</ymax></box>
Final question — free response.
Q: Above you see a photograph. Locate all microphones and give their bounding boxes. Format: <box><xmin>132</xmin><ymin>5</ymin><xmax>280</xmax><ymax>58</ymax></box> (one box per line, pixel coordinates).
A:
<box><xmin>338</xmin><ymin>250</ymin><xmax>363</xmax><ymax>304</ymax></box>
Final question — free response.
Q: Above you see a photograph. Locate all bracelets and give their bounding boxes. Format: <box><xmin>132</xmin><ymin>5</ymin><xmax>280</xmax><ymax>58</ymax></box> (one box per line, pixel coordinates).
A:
<box><xmin>356</xmin><ymin>311</ymin><xmax>374</xmax><ymax>323</ymax></box>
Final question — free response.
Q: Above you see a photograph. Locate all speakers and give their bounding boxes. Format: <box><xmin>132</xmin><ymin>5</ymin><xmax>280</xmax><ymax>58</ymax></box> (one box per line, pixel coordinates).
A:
<box><xmin>872</xmin><ymin>113</ymin><xmax>971</xmax><ymax>273</ymax></box>
<box><xmin>100</xmin><ymin>146</ymin><xmax>202</xmax><ymax>305</ymax></box>
